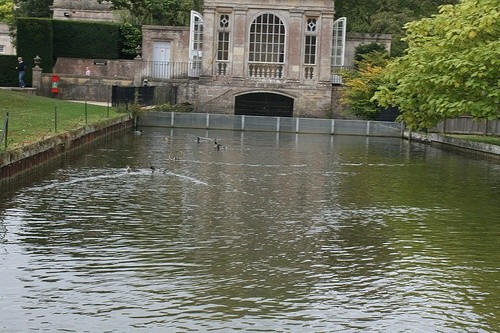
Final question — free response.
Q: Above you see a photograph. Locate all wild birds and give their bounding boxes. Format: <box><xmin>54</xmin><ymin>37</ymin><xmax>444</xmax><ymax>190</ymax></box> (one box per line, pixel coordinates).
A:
<box><xmin>135</xmin><ymin>130</ymin><xmax>144</xmax><ymax>135</ymax></box>
<box><xmin>173</xmin><ymin>156</ymin><xmax>179</xmax><ymax>161</ymax></box>
<box><xmin>197</xmin><ymin>136</ymin><xmax>201</xmax><ymax>141</ymax></box>
<box><xmin>126</xmin><ymin>165</ymin><xmax>139</xmax><ymax>172</ymax></box>
<box><xmin>213</xmin><ymin>137</ymin><xmax>227</xmax><ymax>149</ymax></box>
<box><xmin>149</xmin><ymin>165</ymin><xmax>167</xmax><ymax>174</ymax></box>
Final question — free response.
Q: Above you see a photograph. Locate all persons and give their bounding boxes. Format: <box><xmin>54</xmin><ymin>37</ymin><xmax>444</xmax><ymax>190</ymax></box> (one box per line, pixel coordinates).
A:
<box><xmin>15</xmin><ymin>57</ymin><xmax>27</xmax><ymax>88</ymax></box>
<box><xmin>86</xmin><ymin>67</ymin><xmax>91</xmax><ymax>76</ymax></box>
<box><xmin>280</xmin><ymin>51</ymin><xmax>284</xmax><ymax>62</ymax></box>
